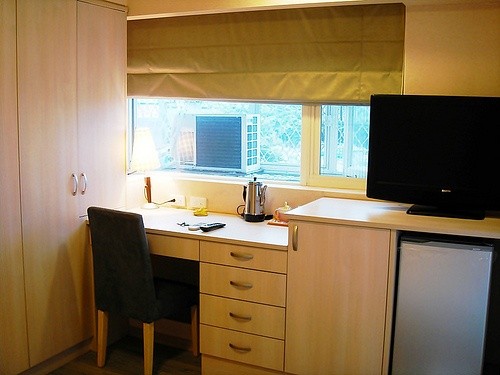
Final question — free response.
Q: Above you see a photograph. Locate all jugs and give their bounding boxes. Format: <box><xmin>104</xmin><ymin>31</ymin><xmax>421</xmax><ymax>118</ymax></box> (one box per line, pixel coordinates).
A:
<box><xmin>274</xmin><ymin>201</ymin><xmax>293</xmax><ymax>222</ymax></box>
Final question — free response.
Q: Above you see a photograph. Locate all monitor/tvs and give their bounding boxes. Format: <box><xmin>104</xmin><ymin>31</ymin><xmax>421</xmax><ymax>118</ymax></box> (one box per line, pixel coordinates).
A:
<box><xmin>365</xmin><ymin>94</ymin><xmax>500</xmax><ymax>220</ymax></box>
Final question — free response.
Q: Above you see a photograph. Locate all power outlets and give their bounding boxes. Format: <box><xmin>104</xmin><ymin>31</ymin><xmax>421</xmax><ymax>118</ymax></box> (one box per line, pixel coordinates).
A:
<box><xmin>170</xmin><ymin>195</ymin><xmax>185</xmax><ymax>206</ymax></box>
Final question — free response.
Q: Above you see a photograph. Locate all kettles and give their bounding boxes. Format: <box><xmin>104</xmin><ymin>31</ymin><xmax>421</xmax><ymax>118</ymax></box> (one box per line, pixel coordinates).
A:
<box><xmin>243</xmin><ymin>176</ymin><xmax>267</xmax><ymax>222</ymax></box>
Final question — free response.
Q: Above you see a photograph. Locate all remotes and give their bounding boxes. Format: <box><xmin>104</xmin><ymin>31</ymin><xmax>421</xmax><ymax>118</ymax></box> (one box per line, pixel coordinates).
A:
<box><xmin>200</xmin><ymin>223</ymin><xmax>226</xmax><ymax>232</ymax></box>
<box><xmin>188</xmin><ymin>223</ymin><xmax>205</xmax><ymax>231</ymax></box>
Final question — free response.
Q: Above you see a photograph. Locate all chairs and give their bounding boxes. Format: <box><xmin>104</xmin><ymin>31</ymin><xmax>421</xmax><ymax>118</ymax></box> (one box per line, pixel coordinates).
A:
<box><xmin>87</xmin><ymin>207</ymin><xmax>200</xmax><ymax>375</ymax></box>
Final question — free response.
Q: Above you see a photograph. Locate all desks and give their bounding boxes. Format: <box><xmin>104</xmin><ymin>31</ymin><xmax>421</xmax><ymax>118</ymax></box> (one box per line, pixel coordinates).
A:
<box><xmin>86</xmin><ymin>205</ymin><xmax>289</xmax><ymax>375</ymax></box>
<box><xmin>284</xmin><ymin>197</ymin><xmax>500</xmax><ymax>239</ymax></box>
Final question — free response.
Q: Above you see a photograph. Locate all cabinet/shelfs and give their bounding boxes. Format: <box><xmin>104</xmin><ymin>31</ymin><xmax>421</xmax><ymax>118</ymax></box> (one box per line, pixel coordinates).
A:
<box><xmin>287</xmin><ymin>219</ymin><xmax>401</xmax><ymax>375</ymax></box>
<box><xmin>200</xmin><ymin>240</ymin><xmax>289</xmax><ymax>375</ymax></box>
<box><xmin>0</xmin><ymin>0</ymin><xmax>130</xmax><ymax>375</ymax></box>
